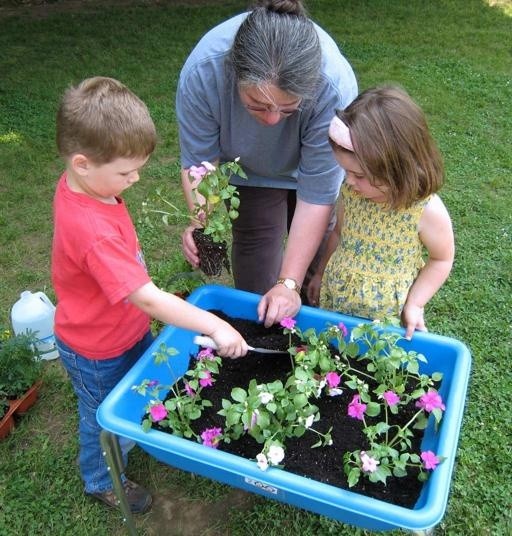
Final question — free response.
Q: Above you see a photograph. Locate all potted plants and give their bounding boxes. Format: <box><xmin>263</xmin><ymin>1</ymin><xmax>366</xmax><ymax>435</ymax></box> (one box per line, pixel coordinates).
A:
<box><xmin>0</xmin><ymin>326</ymin><xmax>48</xmax><ymax>443</ymax></box>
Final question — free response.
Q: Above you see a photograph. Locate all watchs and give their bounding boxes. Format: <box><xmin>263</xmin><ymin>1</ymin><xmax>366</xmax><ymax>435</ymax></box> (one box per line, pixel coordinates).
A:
<box><xmin>276</xmin><ymin>278</ymin><xmax>302</xmax><ymax>295</ymax></box>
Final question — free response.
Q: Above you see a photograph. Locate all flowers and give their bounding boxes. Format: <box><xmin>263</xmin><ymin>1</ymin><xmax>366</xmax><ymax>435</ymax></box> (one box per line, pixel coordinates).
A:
<box><xmin>131</xmin><ymin>316</ymin><xmax>449</xmax><ymax>480</ymax></box>
<box><xmin>133</xmin><ymin>153</ymin><xmax>249</xmax><ymax>248</ymax></box>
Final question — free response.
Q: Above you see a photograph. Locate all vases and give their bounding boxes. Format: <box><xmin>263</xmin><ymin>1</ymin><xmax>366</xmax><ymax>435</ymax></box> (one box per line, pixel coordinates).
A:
<box><xmin>96</xmin><ymin>281</ymin><xmax>472</xmax><ymax>535</ymax></box>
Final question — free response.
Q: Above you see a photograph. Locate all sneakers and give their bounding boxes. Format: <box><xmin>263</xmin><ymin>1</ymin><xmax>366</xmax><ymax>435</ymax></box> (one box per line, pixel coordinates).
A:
<box><xmin>91</xmin><ymin>477</ymin><xmax>154</xmax><ymax>514</ymax></box>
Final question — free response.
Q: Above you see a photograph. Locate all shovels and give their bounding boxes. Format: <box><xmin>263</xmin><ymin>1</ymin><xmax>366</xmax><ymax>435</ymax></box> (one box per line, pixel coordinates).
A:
<box><xmin>194</xmin><ymin>336</ymin><xmax>288</xmax><ymax>354</ymax></box>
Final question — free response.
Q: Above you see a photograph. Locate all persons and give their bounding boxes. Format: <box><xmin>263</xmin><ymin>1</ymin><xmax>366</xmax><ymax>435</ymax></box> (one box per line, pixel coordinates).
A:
<box><xmin>175</xmin><ymin>1</ymin><xmax>359</xmax><ymax>329</ymax></box>
<box><xmin>306</xmin><ymin>87</ymin><xmax>455</xmax><ymax>341</ymax></box>
<box><xmin>51</xmin><ymin>76</ymin><xmax>248</xmax><ymax>514</ymax></box>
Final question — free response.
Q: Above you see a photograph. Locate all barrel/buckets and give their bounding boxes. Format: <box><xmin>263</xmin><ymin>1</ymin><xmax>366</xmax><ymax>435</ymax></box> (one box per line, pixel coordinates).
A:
<box><xmin>11</xmin><ymin>290</ymin><xmax>59</xmax><ymax>361</ymax></box>
